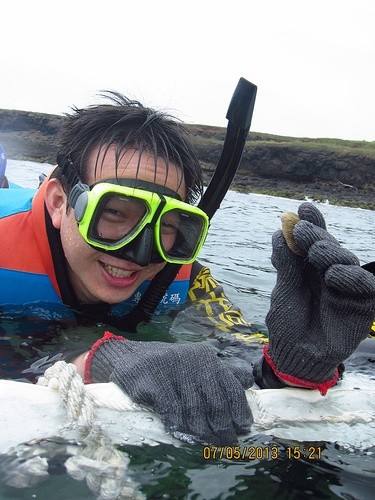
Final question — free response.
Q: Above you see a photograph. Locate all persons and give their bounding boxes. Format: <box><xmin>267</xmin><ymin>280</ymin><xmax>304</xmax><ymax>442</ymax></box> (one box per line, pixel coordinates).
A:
<box><xmin>0</xmin><ymin>86</ymin><xmax>375</xmax><ymax>454</ymax></box>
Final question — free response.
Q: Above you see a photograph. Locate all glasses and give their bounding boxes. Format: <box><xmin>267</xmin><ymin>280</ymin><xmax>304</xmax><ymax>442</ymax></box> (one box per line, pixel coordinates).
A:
<box><xmin>54</xmin><ymin>154</ymin><xmax>210</xmax><ymax>264</ymax></box>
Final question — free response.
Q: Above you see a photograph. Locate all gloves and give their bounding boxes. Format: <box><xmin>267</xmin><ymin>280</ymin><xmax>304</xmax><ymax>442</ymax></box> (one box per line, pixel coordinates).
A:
<box><xmin>262</xmin><ymin>202</ymin><xmax>375</xmax><ymax>395</ymax></box>
<box><xmin>84</xmin><ymin>329</ymin><xmax>255</xmax><ymax>447</ymax></box>
